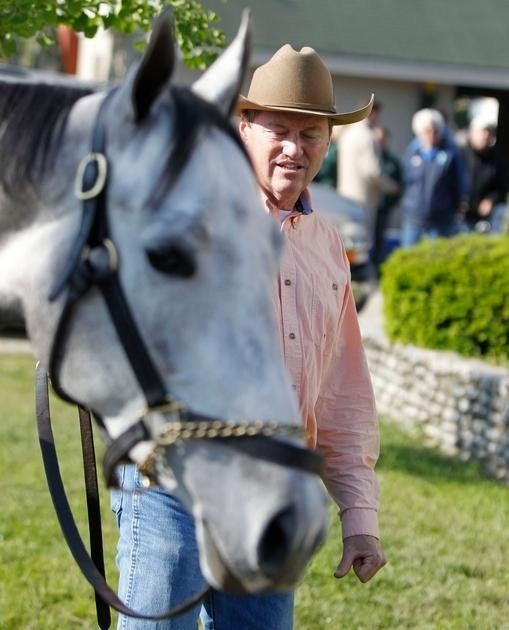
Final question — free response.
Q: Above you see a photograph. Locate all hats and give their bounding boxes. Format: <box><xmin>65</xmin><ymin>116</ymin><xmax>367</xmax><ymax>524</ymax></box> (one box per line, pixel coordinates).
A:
<box><xmin>232</xmin><ymin>44</ymin><xmax>375</xmax><ymax>125</ymax></box>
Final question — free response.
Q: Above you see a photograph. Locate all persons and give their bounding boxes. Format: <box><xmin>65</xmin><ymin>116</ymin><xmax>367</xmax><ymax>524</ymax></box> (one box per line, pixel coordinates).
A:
<box><xmin>104</xmin><ymin>42</ymin><xmax>387</xmax><ymax>630</ymax></box>
<box><xmin>304</xmin><ymin>94</ymin><xmax>508</xmax><ymax>310</ymax></box>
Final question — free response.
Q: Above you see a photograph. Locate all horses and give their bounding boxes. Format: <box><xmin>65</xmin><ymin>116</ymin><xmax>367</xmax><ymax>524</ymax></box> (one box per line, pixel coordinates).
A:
<box><xmin>0</xmin><ymin>4</ymin><xmax>335</xmax><ymax>599</ymax></box>
